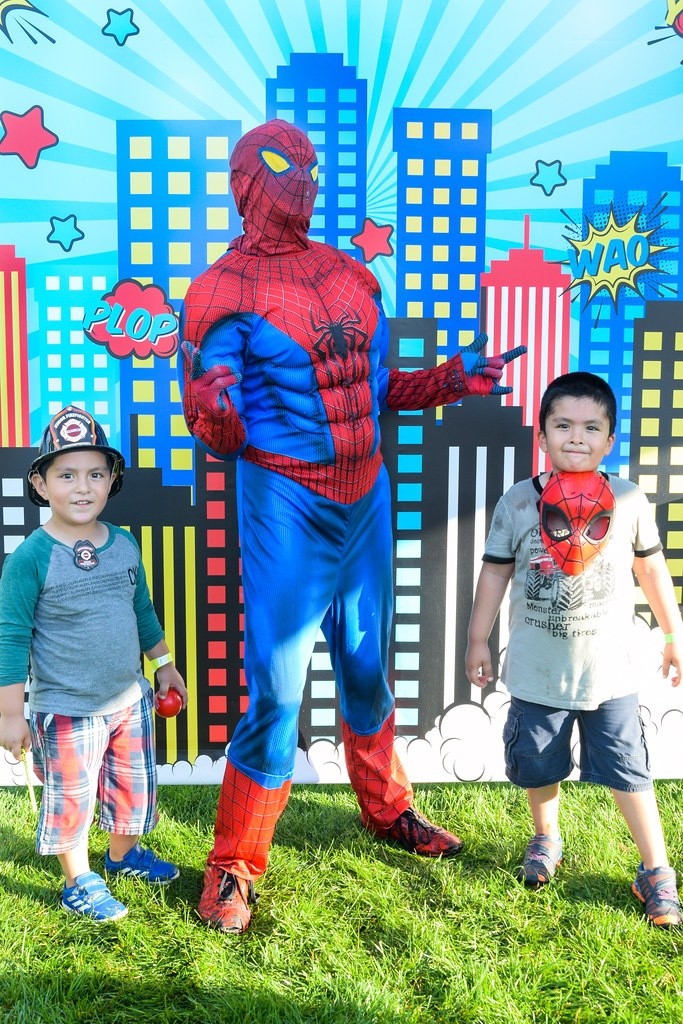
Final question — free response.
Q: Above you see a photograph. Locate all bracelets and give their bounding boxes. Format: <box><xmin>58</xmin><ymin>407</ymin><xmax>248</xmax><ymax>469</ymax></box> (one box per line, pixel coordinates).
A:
<box><xmin>664</xmin><ymin>632</ymin><xmax>683</xmax><ymax>644</ymax></box>
<box><xmin>150</xmin><ymin>653</ymin><xmax>173</xmax><ymax>672</ymax></box>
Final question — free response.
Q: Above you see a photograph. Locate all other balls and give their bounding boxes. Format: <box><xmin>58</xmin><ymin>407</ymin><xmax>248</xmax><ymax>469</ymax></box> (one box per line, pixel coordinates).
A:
<box><xmin>155</xmin><ymin>689</ymin><xmax>182</xmax><ymax>719</ymax></box>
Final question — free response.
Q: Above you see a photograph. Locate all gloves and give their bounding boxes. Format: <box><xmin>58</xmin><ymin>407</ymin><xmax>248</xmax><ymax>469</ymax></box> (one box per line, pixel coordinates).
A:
<box><xmin>461</xmin><ymin>331</ymin><xmax>527</xmax><ymax>395</ymax></box>
<box><xmin>181</xmin><ymin>341</ymin><xmax>243</xmax><ymax>417</ymax></box>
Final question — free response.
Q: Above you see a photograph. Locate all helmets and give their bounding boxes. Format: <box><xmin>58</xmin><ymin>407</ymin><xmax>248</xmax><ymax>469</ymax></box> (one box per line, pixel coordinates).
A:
<box><xmin>27</xmin><ymin>404</ymin><xmax>125</xmax><ymax>506</ymax></box>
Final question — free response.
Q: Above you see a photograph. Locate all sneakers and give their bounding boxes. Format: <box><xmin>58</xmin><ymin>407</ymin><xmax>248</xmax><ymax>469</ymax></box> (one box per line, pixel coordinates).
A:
<box><xmin>519</xmin><ymin>835</ymin><xmax>564</xmax><ymax>884</ymax></box>
<box><xmin>105</xmin><ymin>844</ymin><xmax>180</xmax><ymax>886</ymax></box>
<box><xmin>199</xmin><ymin>859</ymin><xmax>261</xmax><ymax>936</ymax></box>
<box><xmin>632</xmin><ymin>862</ymin><xmax>679</xmax><ymax>927</ymax></box>
<box><xmin>62</xmin><ymin>872</ymin><xmax>128</xmax><ymax>922</ymax></box>
<box><xmin>389</xmin><ymin>805</ymin><xmax>462</xmax><ymax>857</ymax></box>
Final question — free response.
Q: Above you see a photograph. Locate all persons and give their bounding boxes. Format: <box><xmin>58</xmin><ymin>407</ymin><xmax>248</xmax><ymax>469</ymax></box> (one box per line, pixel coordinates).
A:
<box><xmin>0</xmin><ymin>405</ymin><xmax>188</xmax><ymax>923</ymax></box>
<box><xmin>465</xmin><ymin>372</ymin><xmax>682</xmax><ymax>925</ymax></box>
<box><xmin>175</xmin><ymin>117</ymin><xmax>527</xmax><ymax>932</ymax></box>
